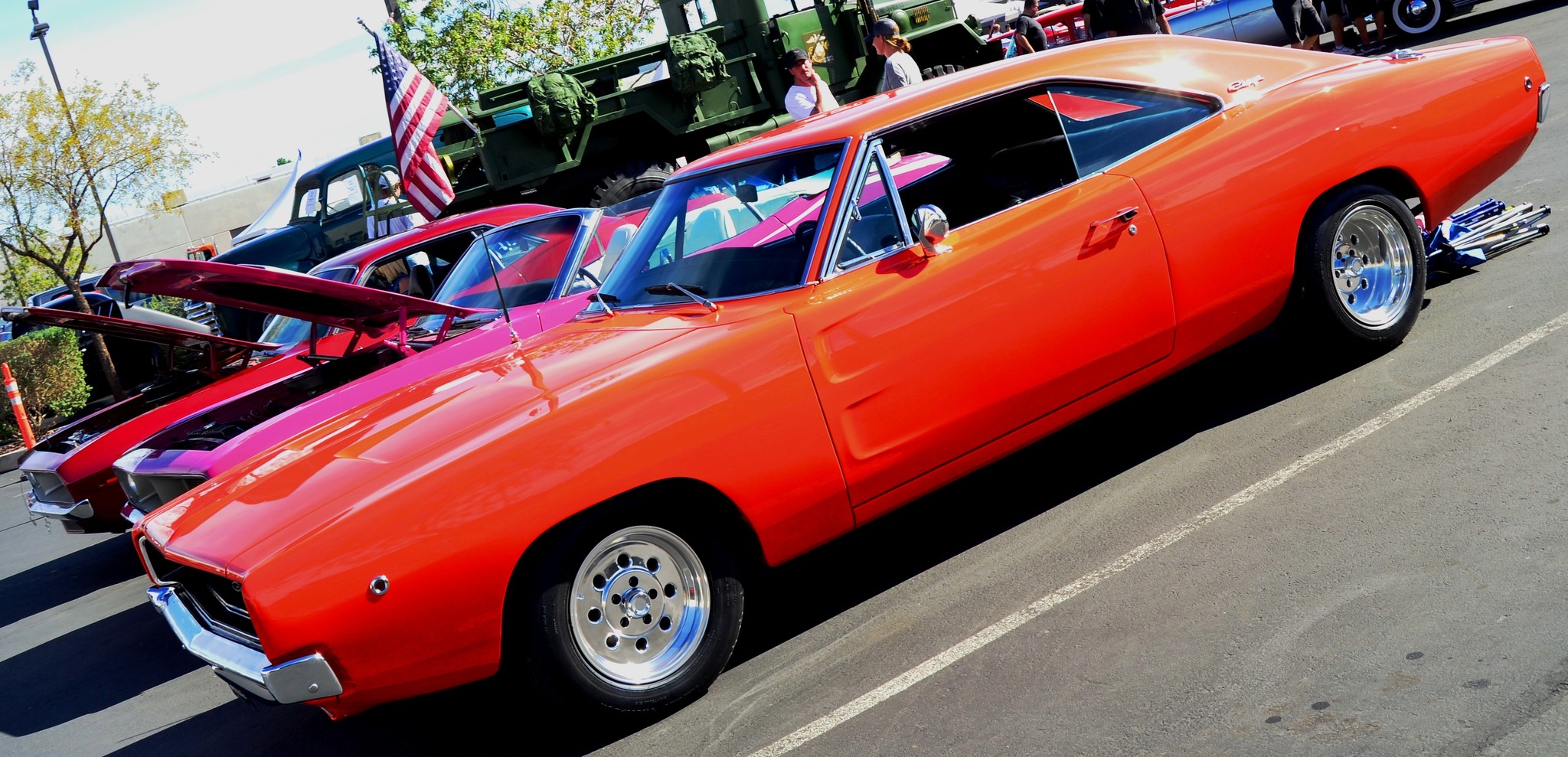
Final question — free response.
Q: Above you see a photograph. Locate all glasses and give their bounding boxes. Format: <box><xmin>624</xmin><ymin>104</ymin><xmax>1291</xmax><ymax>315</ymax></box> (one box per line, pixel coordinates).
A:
<box><xmin>1033</xmin><ymin>5</ymin><xmax>1039</xmax><ymax>9</ymax></box>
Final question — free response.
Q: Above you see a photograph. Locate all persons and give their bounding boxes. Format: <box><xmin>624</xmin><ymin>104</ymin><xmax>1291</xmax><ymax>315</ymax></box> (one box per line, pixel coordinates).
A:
<box><xmin>991</xmin><ymin>23</ymin><xmax>1012</xmax><ymax>41</ymax></box>
<box><xmin>1055</xmin><ymin>0</ymin><xmax>1076</xmax><ymax>9</ymax></box>
<box><xmin>366</xmin><ymin>171</ymin><xmax>436</xmax><ymax>276</ymax></box>
<box><xmin>376</xmin><ymin>258</ymin><xmax>426</xmax><ymax>302</ymax></box>
<box><xmin>1081</xmin><ymin>0</ymin><xmax>1109</xmax><ymax>44</ymax></box>
<box><xmin>784</xmin><ymin>46</ymin><xmax>843</xmax><ymax>175</ymax></box>
<box><xmin>1016</xmin><ymin>0</ymin><xmax>1049</xmax><ymax>56</ymax></box>
<box><xmin>1102</xmin><ymin>0</ymin><xmax>1174</xmax><ymax>39</ymax></box>
<box><xmin>1271</xmin><ymin>0</ymin><xmax>1389</xmax><ymax>61</ymax></box>
<box><xmin>863</xmin><ymin>17</ymin><xmax>924</xmax><ymax>97</ymax></box>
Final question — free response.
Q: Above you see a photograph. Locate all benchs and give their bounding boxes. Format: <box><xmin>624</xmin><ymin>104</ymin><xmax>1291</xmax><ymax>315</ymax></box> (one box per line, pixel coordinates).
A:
<box><xmin>984</xmin><ymin>106</ymin><xmax>1210</xmax><ymax>205</ymax></box>
<box><xmin>728</xmin><ymin>192</ymin><xmax>798</xmax><ymax>235</ymax></box>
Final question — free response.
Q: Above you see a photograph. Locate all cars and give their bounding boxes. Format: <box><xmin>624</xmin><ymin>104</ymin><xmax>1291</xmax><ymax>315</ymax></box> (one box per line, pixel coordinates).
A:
<box><xmin>0</xmin><ymin>0</ymin><xmax>1551</xmax><ymax>723</ymax></box>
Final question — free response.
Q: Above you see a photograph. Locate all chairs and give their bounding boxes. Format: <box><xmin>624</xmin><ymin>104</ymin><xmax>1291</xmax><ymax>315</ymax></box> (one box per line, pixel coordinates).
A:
<box><xmin>408</xmin><ymin>265</ymin><xmax>435</xmax><ymax>299</ymax></box>
<box><xmin>598</xmin><ymin>223</ymin><xmax>637</xmax><ymax>283</ymax></box>
<box><xmin>840</xmin><ymin>213</ymin><xmax>904</xmax><ymax>266</ymax></box>
<box><xmin>683</xmin><ymin>206</ymin><xmax>737</xmax><ymax>255</ymax></box>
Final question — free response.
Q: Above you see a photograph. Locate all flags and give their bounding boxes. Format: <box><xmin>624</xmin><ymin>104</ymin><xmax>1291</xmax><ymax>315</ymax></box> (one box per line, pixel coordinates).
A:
<box><xmin>372</xmin><ymin>33</ymin><xmax>456</xmax><ymax>224</ymax></box>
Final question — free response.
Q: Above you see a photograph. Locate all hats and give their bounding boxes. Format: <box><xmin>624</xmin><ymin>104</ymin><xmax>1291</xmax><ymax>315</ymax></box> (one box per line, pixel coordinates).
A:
<box><xmin>784</xmin><ymin>47</ymin><xmax>808</xmax><ymax>68</ymax></box>
<box><xmin>863</xmin><ymin>18</ymin><xmax>899</xmax><ymax>42</ymax></box>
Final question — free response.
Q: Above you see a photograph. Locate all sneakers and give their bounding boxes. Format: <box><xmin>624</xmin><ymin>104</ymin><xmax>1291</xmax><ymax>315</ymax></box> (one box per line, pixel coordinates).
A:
<box><xmin>1375</xmin><ymin>42</ymin><xmax>1386</xmax><ymax>50</ymax></box>
<box><xmin>1355</xmin><ymin>44</ymin><xmax>1375</xmax><ymax>56</ymax></box>
<box><xmin>1333</xmin><ymin>46</ymin><xmax>1355</xmax><ymax>55</ymax></box>
